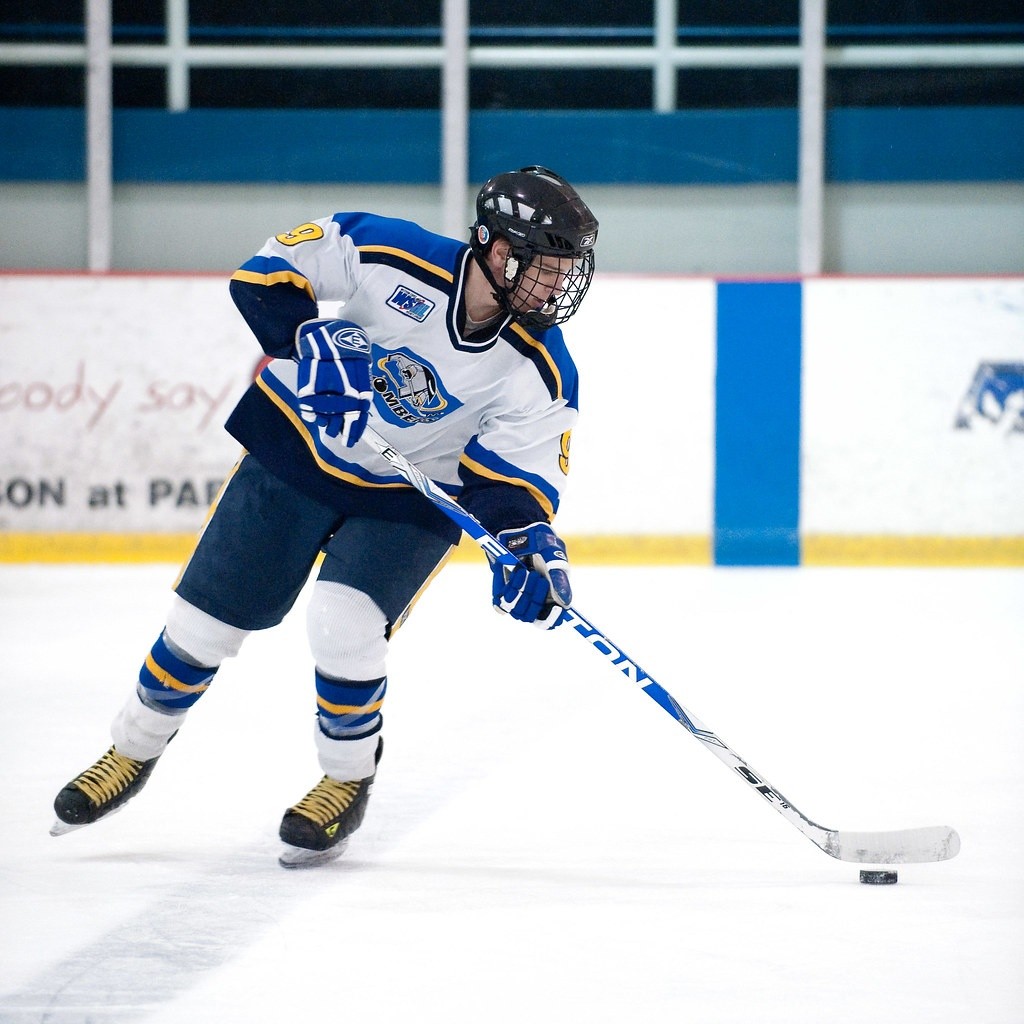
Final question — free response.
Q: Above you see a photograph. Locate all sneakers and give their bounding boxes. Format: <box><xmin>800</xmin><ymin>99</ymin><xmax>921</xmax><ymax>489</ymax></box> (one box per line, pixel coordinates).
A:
<box><xmin>49</xmin><ymin>748</ymin><xmax>162</xmax><ymax>836</ymax></box>
<box><xmin>278</xmin><ymin>774</ymin><xmax>376</xmax><ymax>869</ymax></box>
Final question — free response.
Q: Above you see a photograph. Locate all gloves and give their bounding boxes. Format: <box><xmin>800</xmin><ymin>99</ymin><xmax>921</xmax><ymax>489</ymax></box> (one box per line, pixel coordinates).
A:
<box><xmin>492</xmin><ymin>522</ymin><xmax>572</xmax><ymax>630</ymax></box>
<box><xmin>293</xmin><ymin>318</ymin><xmax>375</xmax><ymax>446</ymax></box>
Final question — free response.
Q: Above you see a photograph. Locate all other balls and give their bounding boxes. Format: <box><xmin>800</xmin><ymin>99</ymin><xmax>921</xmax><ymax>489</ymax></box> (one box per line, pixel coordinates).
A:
<box><xmin>859</xmin><ymin>869</ymin><xmax>898</xmax><ymax>886</ymax></box>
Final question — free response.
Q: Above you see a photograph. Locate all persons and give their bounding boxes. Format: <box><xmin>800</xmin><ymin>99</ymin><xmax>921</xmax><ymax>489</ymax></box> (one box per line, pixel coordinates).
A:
<box><xmin>56</xmin><ymin>161</ymin><xmax>598</xmax><ymax>851</ymax></box>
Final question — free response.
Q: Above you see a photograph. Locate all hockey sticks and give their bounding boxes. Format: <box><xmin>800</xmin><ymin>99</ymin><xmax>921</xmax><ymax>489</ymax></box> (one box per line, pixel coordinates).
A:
<box><xmin>350</xmin><ymin>417</ymin><xmax>969</xmax><ymax>893</ymax></box>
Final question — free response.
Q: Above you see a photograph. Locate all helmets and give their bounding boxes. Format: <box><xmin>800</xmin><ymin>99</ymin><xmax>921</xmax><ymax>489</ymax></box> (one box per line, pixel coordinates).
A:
<box><xmin>468</xmin><ymin>164</ymin><xmax>598</xmax><ymax>260</ymax></box>
